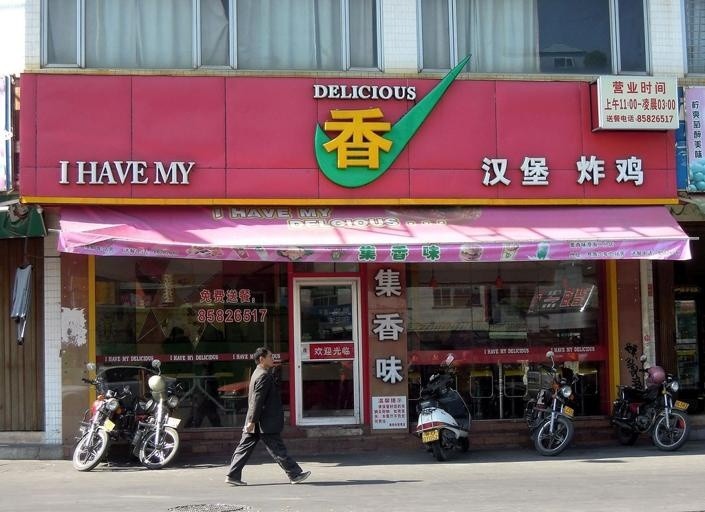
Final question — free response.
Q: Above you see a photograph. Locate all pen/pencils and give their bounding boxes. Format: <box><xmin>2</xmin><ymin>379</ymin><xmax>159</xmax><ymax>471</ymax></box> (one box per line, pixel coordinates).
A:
<box><xmin>217</xmin><ymin>357</ymin><xmax>342</xmax><ymax>415</ymax></box>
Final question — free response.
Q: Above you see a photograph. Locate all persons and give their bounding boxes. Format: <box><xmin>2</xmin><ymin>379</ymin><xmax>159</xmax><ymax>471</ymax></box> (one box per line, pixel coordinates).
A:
<box><xmin>225</xmin><ymin>345</ymin><xmax>312</xmax><ymax>487</ymax></box>
<box><xmin>163</xmin><ymin>325</ymin><xmax>194</xmax><ymax>401</ymax></box>
<box><xmin>185</xmin><ymin>360</ymin><xmax>229</xmax><ymax>427</ymax></box>
<box><xmin>440</xmin><ymin>342</ymin><xmax>485</xmax><ymax>411</ymax></box>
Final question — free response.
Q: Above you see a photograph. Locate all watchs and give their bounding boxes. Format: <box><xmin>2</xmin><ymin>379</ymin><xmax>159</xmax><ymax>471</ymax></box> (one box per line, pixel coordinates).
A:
<box><xmin>129</xmin><ymin>359</ymin><xmax>188</xmax><ymax>470</ymax></box>
<box><xmin>609</xmin><ymin>354</ymin><xmax>689</xmax><ymax>452</ymax></box>
<box><xmin>72</xmin><ymin>361</ymin><xmax>147</xmax><ymax>472</ymax></box>
<box><xmin>412</xmin><ymin>353</ymin><xmax>471</xmax><ymax>463</ymax></box>
<box><xmin>522</xmin><ymin>350</ymin><xmax>584</xmax><ymax>456</ymax></box>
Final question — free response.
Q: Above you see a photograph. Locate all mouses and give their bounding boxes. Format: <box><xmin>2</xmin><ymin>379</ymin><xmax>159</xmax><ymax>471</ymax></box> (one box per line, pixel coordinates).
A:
<box><xmin>18</xmin><ymin>254</ymin><xmax>30</xmax><ymax>267</ymax></box>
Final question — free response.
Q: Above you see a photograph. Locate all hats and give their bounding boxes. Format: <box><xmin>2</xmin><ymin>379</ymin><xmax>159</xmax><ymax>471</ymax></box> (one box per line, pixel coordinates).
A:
<box><xmin>97</xmin><ymin>364</ymin><xmax>180</xmax><ymax>412</ymax></box>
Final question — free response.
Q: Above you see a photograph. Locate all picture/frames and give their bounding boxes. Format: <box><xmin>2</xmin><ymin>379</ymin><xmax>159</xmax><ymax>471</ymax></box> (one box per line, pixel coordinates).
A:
<box><xmin>176</xmin><ymin>376</ymin><xmax>227</xmax><ymax>414</ymax></box>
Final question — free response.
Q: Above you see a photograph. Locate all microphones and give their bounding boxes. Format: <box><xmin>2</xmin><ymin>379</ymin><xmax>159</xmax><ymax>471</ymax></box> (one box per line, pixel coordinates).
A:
<box><xmin>409</xmin><ymin>367</ymin><xmax>598</xmax><ymax>397</ymax></box>
<box><xmin>219</xmin><ymin>383</ymin><xmax>248</xmax><ymax>419</ymax></box>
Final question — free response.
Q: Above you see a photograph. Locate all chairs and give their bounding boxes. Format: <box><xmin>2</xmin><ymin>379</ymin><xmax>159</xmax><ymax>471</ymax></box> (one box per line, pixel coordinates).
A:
<box><xmin>291</xmin><ymin>471</ymin><xmax>311</xmax><ymax>485</ymax></box>
<box><xmin>225</xmin><ymin>477</ymin><xmax>247</xmax><ymax>486</ymax></box>
